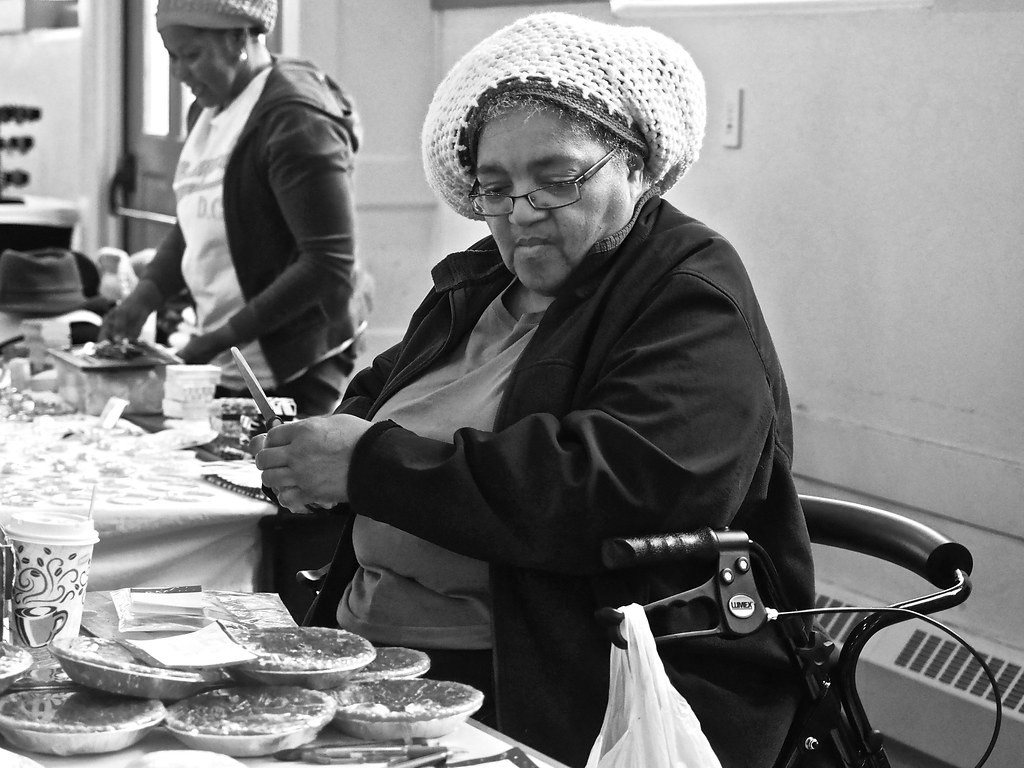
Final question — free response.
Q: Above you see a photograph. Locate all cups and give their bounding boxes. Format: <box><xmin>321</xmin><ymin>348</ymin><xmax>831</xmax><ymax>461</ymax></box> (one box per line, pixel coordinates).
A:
<box><xmin>5</xmin><ymin>512</ymin><xmax>99</xmax><ymax>648</ymax></box>
<box><xmin>15</xmin><ymin>606</ymin><xmax>68</xmax><ymax>648</ymax></box>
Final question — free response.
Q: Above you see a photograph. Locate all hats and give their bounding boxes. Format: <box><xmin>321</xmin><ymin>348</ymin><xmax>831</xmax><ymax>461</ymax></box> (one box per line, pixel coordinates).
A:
<box><xmin>1</xmin><ymin>247</ymin><xmax>117</xmax><ymax>316</ymax></box>
<box><xmin>155</xmin><ymin>0</ymin><xmax>278</xmax><ymax>34</ymax></box>
<box><xmin>421</xmin><ymin>11</ymin><xmax>709</xmax><ymax>220</ymax></box>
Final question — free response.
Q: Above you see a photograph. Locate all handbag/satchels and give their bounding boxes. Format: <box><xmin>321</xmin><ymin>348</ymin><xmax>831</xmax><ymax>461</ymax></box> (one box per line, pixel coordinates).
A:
<box><xmin>581</xmin><ymin>601</ymin><xmax>721</xmax><ymax>768</ymax></box>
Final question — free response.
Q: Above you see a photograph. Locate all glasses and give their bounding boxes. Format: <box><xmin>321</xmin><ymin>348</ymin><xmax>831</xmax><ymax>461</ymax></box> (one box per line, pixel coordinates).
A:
<box><xmin>467</xmin><ymin>143</ymin><xmax>618</xmax><ymax>217</ymax></box>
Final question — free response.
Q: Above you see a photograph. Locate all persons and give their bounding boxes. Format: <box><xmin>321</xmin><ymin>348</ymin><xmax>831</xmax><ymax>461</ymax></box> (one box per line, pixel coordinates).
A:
<box><xmin>97</xmin><ymin>1</ymin><xmax>373</xmax><ymax>630</ymax></box>
<box><xmin>244</xmin><ymin>7</ymin><xmax>817</xmax><ymax>768</ymax></box>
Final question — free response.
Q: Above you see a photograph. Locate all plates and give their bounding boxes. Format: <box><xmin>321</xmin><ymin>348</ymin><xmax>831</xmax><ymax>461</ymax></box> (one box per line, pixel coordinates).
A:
<box><xmin>331</xmin><ymin>679</ymin><xmax>482</xmax><ymax>740</ymax></box>
<box><xmin>349</xmin><ymin>646</ymin><xmax>431</xmax><ymax>682</ymax></box>
<box><xmin>50</xmin><ymin>636</ymin><xmax>218</xmax><ymax>700</ymax></box>
<box><xmin>0</xmin><ymin>643</ymin><xmax>33</xmax><ymax>693</ymax></box>
<box><xmin>239</xmin><ymin>627</ymin><xmax>375</xmax><ymax>689</ymax></box>
<box><xmin>167</xmin><ymin>687</ymin><xmax>335</xmax><ymax>757</ymax></box>
<box><xmin>0</xmin><ymin>690</ymin><xmax>167</xmax><ymax>755</ymax></box>
